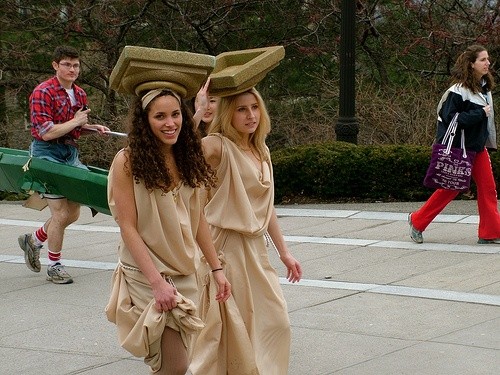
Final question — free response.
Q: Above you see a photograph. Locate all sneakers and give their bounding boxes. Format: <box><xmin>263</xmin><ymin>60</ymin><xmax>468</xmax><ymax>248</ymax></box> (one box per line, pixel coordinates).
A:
<box><xmin>46</xmin><ymin>263</ymin><xmax>73</xmax><ymax>284</ymax></box>
<box><xmin>477</xmin><ymin>237</ymin><xmax>500</xmax><ymax>244</ymax></box>
<box><xmin>408</xmin><ymin>212</ymin><xmax>423</xmax><ymax>243</ymax></box>
<box><xmin>18</xmin><ymin>232</ymin><xmax>44</xmax><ymax>272</ymax></box>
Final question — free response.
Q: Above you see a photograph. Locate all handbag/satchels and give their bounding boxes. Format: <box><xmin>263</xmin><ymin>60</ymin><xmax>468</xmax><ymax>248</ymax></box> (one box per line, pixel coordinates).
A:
<box><xmin>422</xmin><ymin>113</ymin><xmax>476</xmax><ymax>192</ymax></box>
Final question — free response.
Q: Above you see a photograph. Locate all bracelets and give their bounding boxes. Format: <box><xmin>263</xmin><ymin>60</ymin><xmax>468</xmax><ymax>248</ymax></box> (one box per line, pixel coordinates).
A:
<box><xmin>211</xmin><ymin>268</ymin><xmax>223</xmax><ymax>272</ymax></box>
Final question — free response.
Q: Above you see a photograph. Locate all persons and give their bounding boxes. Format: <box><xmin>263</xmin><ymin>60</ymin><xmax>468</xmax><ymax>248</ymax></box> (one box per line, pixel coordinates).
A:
<box><xmin>108</xmin><ymin>88</ymin><xmax>231</xmax><ymax>375</ymax></box>
<box><xmin>193</xmin><ymin>76</ymin><xmax>303</xmax><ymax>375</ymax></box>
<box><xmin>18</xmin><ymin>45</ymin><xmax>110</xmax><ymax>284</ymax></box>
<box><xmin>407</xmin><ymin>45</ymin><xmax>500</xmax><ymax>245</ymax></box>
<box><xmin>190</xmin><ymin>92</ymin><xmax>219</xmax><ymax>138</ymax></box>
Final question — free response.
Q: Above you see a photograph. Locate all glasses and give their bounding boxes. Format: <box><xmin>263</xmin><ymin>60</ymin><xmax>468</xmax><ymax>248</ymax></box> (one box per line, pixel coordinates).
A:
<box><xmin>57</xmin><ymin>62</ymin><xmax>80</xmax><ymax>68</ymax></box>
